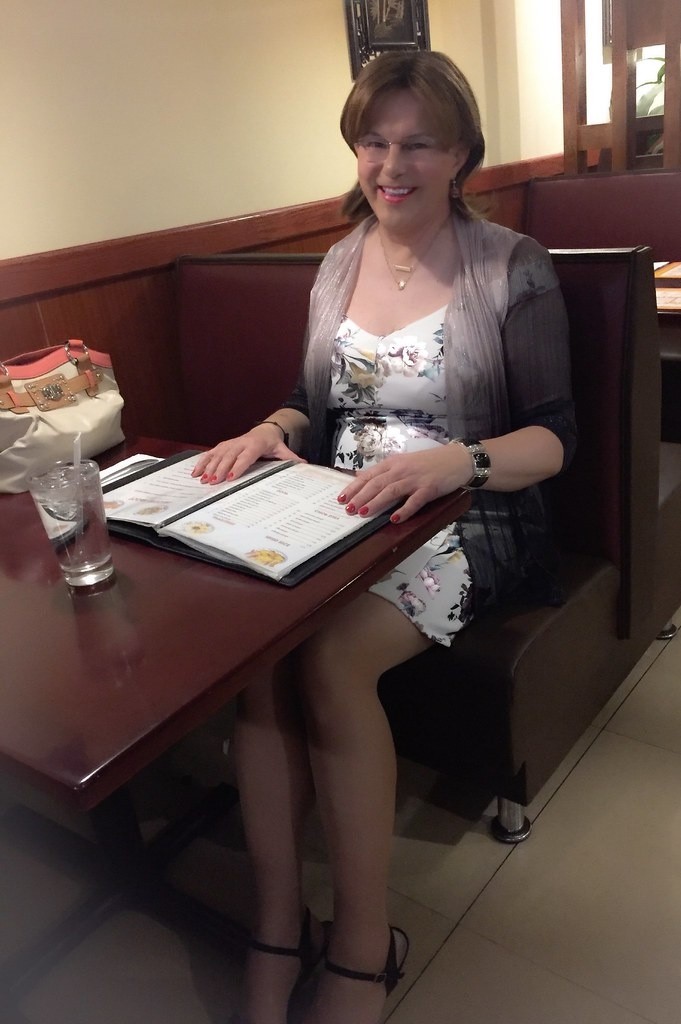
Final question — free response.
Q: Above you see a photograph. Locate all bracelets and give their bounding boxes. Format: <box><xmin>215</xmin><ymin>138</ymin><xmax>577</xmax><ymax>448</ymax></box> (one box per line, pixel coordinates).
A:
<box><xmin>450</xmin><ymin>434</ymin><xmax>492</xmax><ymax>501</ymax></box>
<box><xmin>257</xmin><ymin>421</ymin><xmax>290</xmax><ymax>449</ymax></box>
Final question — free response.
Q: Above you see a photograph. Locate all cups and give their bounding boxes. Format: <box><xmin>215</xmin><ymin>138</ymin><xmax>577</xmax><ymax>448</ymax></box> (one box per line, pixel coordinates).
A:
<box><xmin>25</xmin><ymin>458</ymin><xmax>117</xmax><ymax>597</ymax></box>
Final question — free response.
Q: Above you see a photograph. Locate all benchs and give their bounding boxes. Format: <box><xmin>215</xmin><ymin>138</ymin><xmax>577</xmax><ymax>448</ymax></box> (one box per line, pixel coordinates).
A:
<box><xmin>168</xmin><ymin>244</ymin><xmax>681</xmax><ymax>843</ymax></box>
<box><xmin>525</xmin><ymin>169</ymin><xmax>681</xmax><ymax>264</ymax></box>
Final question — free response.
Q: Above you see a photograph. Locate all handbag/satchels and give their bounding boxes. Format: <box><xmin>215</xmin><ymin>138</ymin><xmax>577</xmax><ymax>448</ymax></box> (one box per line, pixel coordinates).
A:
<box><xmin>0</xmin><ymin>339</ymin><xmax>127</xmax><ymax>494</ymax></box>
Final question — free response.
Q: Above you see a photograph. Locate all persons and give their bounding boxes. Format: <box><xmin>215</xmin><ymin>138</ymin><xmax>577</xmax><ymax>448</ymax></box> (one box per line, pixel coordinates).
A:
<box><xmin>189</xmin><ymin>53</ymin><xmax>581</xmax><ymax>1024</ymax></box>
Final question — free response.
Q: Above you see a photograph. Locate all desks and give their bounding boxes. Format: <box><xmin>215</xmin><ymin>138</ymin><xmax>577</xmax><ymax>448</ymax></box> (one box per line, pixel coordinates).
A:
<box><xmin>654</xmin><ymin>278</ymin><xmax>680</xmax><ymax>445</ymax></box>
<box><xmin>0</xmin><ymin>439</ymin><xmax>473</xmax><ymax>1024</ymax></box>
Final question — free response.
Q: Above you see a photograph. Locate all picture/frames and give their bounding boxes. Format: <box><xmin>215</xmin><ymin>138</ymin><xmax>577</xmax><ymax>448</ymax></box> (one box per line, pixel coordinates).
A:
<box><xmin>344</xmin><ymin>0</ymin><xmax>431</xmax><ymax>83</ymax></box>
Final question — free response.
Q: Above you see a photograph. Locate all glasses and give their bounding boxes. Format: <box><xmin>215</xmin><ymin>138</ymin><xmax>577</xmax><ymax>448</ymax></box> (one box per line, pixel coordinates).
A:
<box><xmin>353</xmin><ymin>135</ymin><xmax>459</xmax><ymax>163</ymax></box>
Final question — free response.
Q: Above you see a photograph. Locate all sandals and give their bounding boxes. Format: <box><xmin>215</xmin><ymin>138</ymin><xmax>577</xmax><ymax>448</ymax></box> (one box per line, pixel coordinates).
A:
<box><xmin>310</xmin><ymin>924</ymin><xmax>409</xmax><ymax>1024</ymax></box>
<box><xmin>234</xmin><ymin>904</ymin><xmax>332</xmax><ymax>1024</ymax></box>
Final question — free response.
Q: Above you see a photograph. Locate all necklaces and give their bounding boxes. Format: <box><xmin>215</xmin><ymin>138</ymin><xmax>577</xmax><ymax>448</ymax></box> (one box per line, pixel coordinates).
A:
<box><xmin>376</xmin><ymin>212</ymin><xmax>451</xmax><ymax>290</ymax></box>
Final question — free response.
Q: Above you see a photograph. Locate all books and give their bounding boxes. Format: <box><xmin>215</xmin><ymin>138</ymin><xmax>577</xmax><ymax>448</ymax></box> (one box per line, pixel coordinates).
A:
<box><xmin>103</xmin><ymin>451</ymin><xmax>408</xmax><ymax>588</ymax></box>
